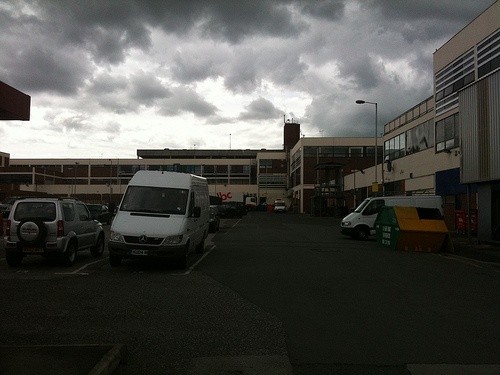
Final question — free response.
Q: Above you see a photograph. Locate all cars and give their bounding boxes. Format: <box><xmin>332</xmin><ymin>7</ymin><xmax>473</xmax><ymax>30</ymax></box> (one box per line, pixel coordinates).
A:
<box><xmin>210</xmin><ymin>204</ymin><xmax>221</xmax><ymax>231</ymax></box>
<box><xmin>84</xmin><ymin>202</ymin><xmax>110</xmax><ymax>223</ymax></box>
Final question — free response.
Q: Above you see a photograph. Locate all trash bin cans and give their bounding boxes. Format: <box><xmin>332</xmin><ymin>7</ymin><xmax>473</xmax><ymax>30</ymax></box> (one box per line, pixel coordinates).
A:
<box><xmin>265</xmin><ymin>203</ymin><xmax>273</xmax><ymax>211</ymax></box>
<box><xmin>373</xmin><ymin>205</ymin><xmax>450</xmax><ymax>253</ymax></box>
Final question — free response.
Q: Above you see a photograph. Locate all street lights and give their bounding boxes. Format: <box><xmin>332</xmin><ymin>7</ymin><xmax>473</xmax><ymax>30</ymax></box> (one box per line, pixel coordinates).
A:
<box><xmin>356</xmin><ymin>99</ymin><xmax>378</xmax><ymax>197</ymax></box>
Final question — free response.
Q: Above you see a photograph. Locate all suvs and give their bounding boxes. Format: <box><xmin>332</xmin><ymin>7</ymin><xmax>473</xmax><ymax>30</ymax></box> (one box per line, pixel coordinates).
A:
<box><xmin>4</xmin><ymin>197</ymin><xmax>105</xmax><ymax>266</ymax></box>
<box><xmin>218</xmin><ymin>201</ymin><xmax>249</xmax><ymax>218</ymax></box>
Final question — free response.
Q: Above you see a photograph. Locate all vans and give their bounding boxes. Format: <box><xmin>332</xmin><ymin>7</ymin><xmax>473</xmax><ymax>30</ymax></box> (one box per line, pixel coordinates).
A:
<box><xmin>107</xmin><ymin>168</ymin><xmax>212</xmax><ymax>267</ymax></box>
<box><xmin>273</xmin><ymin>199</ymin><xmax>287</xmax><ymax>213</ymax></box>
<box><xmin>340</xmin><ymin>194</ymin><xmax>444</xmax><ymax>240</ymax></box>
